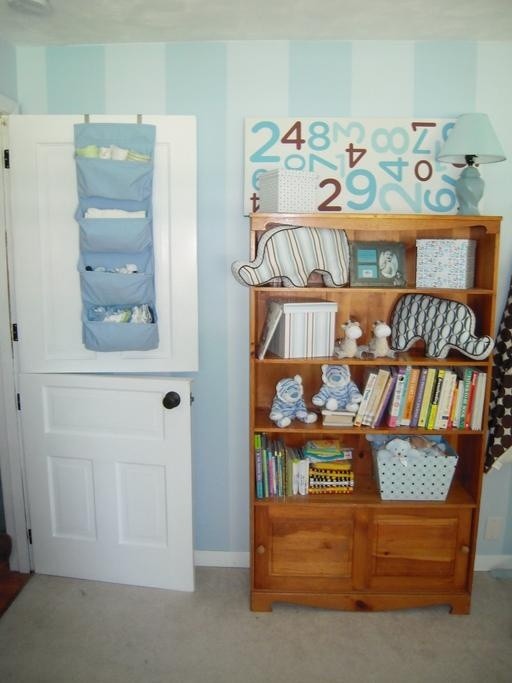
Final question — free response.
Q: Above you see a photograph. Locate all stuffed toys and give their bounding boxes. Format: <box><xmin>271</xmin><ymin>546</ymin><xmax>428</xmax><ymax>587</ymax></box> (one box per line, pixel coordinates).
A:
<box><xmin>312</xmin><ymin>363</ymin><xmax>363</xmax><ymax>412</ymax></box>
<box><xmin>333</xmin><ymin>319</ymin><xmax>369</xmax><ymax>359</ymax></box>
<box><xmin>390</xmin><ymin>294</ymin><xmax>495</xmax><ymax>361</ymax></box>
<box><xmin>364</xmin><ymin>432</ymin><xmax>446</xmax><ymax>466</ymax></box>
<box><xmin>268</xmin><ymin>374</ymin><xmax>318</xmax><ymax>428</ymax></box>
<box><xmin>230</xmin><ymin>223</ymin><xmax>351</xmax><ymax>288</ymax></box>
<box><xmin>360</xmin><ymin>320</ymin><xmax>399</xmax><ymax>359</ymax></box>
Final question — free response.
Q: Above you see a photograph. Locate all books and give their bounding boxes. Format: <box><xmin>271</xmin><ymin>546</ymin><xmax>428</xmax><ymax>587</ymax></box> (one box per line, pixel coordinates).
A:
<box><xmin>252</xmin><ymin>432</ymin><xmax>355</xmax><ymax>500</ymax></box>
<box><xmin>321</xmin><ymin>365</ymin><xmax>487</xmax><ymax>431</ymax></box>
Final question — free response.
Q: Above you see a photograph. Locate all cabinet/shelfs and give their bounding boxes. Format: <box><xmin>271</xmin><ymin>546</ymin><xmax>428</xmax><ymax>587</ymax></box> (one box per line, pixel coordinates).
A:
<box><xmin>251</xmin><ymin>217</ymin><xmax>502</xmax><ymax>504</ymax></box>
<box><xmin>254</xmin><ymin>501</ymin><xmax>471</xmax><ymax>613</ymax></box>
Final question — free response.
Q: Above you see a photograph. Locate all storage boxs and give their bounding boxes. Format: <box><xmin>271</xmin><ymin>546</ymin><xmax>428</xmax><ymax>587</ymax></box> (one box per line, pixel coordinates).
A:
<box><xmin>414</xmin><ymin>237</ymin><xmax>477</xmax><ymax>291</ymax></box>
<box><xmin>268</xmin><ymin>293</ymin><xmax>336</xmax><ymax>359</ymax></box>
<box><xmin>373</xmin><ymin>433</ymin><xmax>458</xmax><ymax>502</ymax></box>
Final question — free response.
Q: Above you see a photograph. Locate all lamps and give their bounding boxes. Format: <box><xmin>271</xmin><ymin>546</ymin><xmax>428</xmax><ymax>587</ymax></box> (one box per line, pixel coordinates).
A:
<box><xmin>436</xmin><ymin>112</ymin><xmax>507</xmax><ymax>216</ymax></box>
